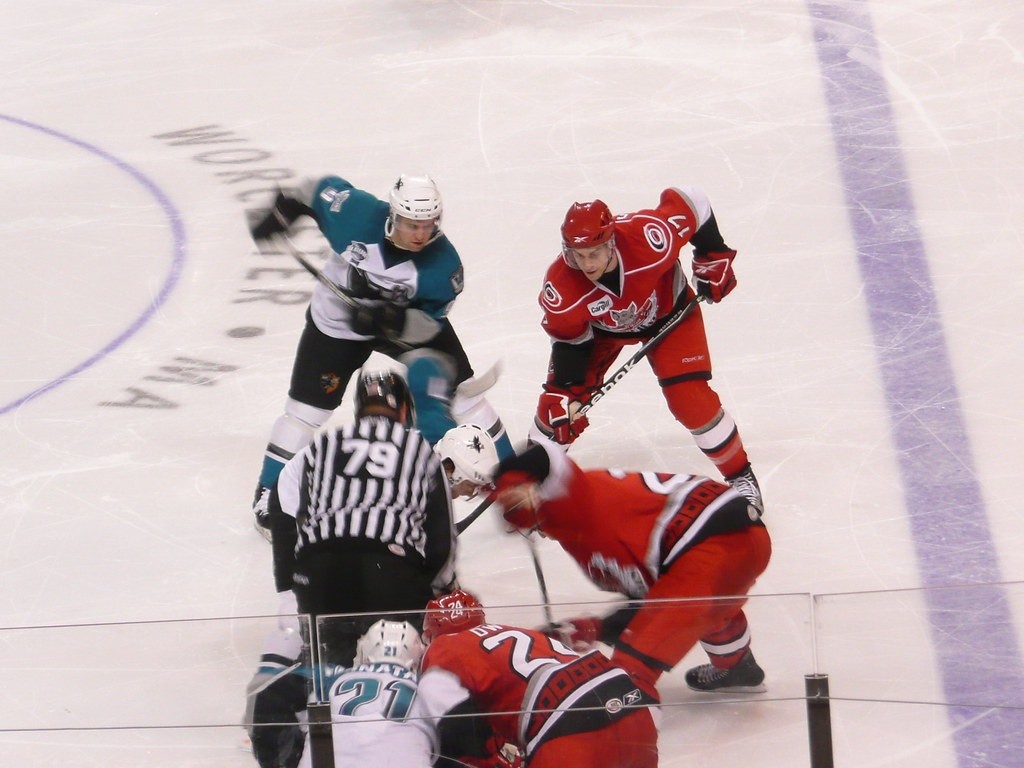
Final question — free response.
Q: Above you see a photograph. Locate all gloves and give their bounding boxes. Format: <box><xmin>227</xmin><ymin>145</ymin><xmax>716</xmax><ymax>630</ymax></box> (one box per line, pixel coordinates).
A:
<box><xmin>350</xmin><ymin>302</ymin><xmax>406</xmax><ymax>339</ymax></box>
<box><xmin>252</xmin><ymin>723</ymin><xmax>305</xmax><ymax>767</ymax></box>
<box><xmin>693</xmin><ymin>248</ymin><xmax>738</xmax><ymax>303</ymax></box>
<box><xmin>550</xmin><ymin>395</ymin><xmax>588</xmax><ymax>447</ymax></box>
<box><xmin>252</xmin><ymin>196</ymin><xmax>297</xmax><ymax>243</ymax></box>
<box><xmin>548</xmin><ymin>615</ymin><xmax>600</xmax><ymax>643</ymax></box>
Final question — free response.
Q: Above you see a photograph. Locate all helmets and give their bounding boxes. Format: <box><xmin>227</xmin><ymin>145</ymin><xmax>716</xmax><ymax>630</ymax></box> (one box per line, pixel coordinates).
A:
<box><xmin>424</xmin><ymin>590</ymin><xmax>484</xmax><ymax>639</ymax></box>
<box><xmin>390</xmin><ymin>170</ymin><xmax>442</xmax><ymax>221</ymax></box>
<box><xmin>562</xmin><ymin>200</ymin><xmax>615</xmax><ymax>248</ymax></box>
<box><xmin>496</xmin><ymin>472</ymin><xmax>541</xmax><ymax>528</ymax></box>
<box><xmin>356</xmin><ymin>619</ymin><xmax>424</xmax><ymax>671</ymax></box>
<box><xmin>433</xmin><ymin>424</ymin><xmax>497</xmax><ymax>489</ymax></box>
<box><xmin>356</xmin><ymin>370</ymin><xmax>416</xmax><ymax>412</ymax></box>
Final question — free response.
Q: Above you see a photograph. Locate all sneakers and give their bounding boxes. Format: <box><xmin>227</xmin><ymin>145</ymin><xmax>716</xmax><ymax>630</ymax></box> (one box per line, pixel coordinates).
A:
<box><xmin>729</xmin><ymin>465</ymin><xmax>764</xmax><ymax>515</ymax></box>
<box><xmin>684</xmin><ymin>650</ymin><xmax>765</xmax><ymax>692</ymax></box>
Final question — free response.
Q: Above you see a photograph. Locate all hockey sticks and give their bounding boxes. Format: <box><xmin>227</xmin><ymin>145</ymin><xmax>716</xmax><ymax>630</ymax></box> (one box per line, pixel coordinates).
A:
<box><xmin>284</xmin><ymin>235</ymin><xmax>506</xmax><ymax>400</ymax></box>
<box><xmin>526</xmin><ymin>534</ymin><xmax>553</xmax><ymax>625</ymax></box>
<box><xmin>453</xmin><ymin>295</ymin><xmax>705</xmax><ymax>534</ymax></box>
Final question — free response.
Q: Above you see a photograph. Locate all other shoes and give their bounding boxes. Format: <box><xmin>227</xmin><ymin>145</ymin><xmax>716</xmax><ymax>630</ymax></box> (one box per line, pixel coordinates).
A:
<box><xmin>253</xmin><ymin>485</ymin><xmax>272</xmax><ymax>541</ymax></box>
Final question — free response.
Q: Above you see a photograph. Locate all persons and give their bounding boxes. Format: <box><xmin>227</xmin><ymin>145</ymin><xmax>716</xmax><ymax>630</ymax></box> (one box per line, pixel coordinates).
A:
<box><xmin>241</xmin><ymin>422</ymin><xmax>501</xmax><ymax>768</ymax></box>
<box><xmin>479</xmin><ymin>435</ymin><xmax>771</xmax><ymax>737</ymax></box>
<box><xmin>504</xmin><ymin>186</ymin><xmax>764</xmax><ymax>533</ymax></box>
<box><xmin>417</xmin><ymin>589</ymin><xmax>659</xmax><ymax>768</ymax></box>
<box><xmin>269</xmin><ymin>367</ymin><xmax>458</xmax><ymax>671</ymax></box>
<box><xmin>246</xmin><ymin>173</ymin><xmax>537</xmax><ymax>544</ymax></box>
